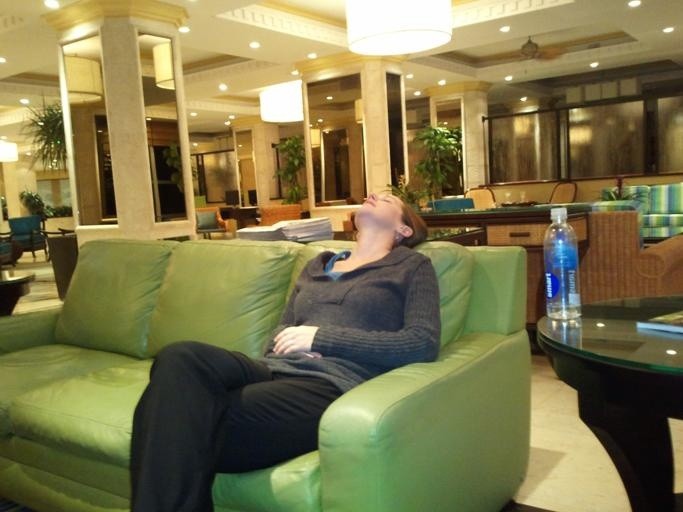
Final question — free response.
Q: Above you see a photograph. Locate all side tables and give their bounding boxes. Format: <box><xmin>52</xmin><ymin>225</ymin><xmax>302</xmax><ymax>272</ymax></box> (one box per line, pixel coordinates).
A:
<box><xmin>537</xmin><ymin>294</ymin><xmax>682</xmax><ymax>512</ymax></box>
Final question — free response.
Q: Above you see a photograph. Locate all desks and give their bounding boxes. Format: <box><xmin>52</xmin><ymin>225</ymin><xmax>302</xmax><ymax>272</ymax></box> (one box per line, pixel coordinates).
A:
<box><xmin>342</xmin><ymin>201</ymin><xmax>591</xmax><ymax>354</ymax></box>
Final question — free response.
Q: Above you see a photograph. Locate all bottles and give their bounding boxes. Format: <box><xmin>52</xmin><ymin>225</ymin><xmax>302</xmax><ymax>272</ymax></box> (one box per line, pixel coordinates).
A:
<box><xmin>543</xmin><ymin>208</ymin><xmax>581</xmax><ymax>321</ymax></box>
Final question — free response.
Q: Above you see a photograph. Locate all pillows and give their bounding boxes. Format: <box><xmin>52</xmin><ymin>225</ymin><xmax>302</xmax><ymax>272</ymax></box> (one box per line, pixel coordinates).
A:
<box><xmin>265</xmin><ymin>238</ymin><xmax>472</xmax><ymax>357</ymax></box>
<box><xmin>52</xmin><ymin>238</ymin><xmax>179</xmax><ymax>360</ymax></box>
<box><xmin>145</xmin><ymin>238</ymin><xmax>300</xmax><ymax>365</ymax></box>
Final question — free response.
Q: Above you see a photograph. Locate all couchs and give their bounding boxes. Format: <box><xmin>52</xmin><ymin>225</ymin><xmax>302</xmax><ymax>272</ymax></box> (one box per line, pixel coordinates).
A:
<box><xmin>0</xmin><ymin>237</ymin><xmax>532</xmax><ymax>512</ymax></box>
<box><xmin>577</xmin><ymin>200</ymin><xmax>683</xmax><ymax>308</ymax></box>
<box><xmin>591</xmin><ymin>181</ymin><xmax>682</xmax><ymax>248</ymax></box>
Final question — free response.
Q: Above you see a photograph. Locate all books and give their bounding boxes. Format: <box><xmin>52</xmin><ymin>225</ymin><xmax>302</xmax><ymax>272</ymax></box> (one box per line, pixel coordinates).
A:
<box><xmin>637</xmin><ymin>311</ymin><xmax>683</xmax><ymax>335</ymax></box>
<box><xmin>236</xmin><ymin>218</ymin><xmax>334</xmax><ymax>244</ymax></box>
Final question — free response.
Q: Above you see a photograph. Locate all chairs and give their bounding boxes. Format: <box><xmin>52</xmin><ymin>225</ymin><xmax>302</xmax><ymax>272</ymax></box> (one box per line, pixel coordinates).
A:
<box><xmin>195</xmin><ymin>206</ymin><xmax>236</xmax><ymax>240</ymax></box>
<box><xmin>259</xmin><ymin>204</ymin><xmax>301</xmax><ymax>226</ymax></box>
<box><xmin>0</xmin><ymin>213</ymin><xmax>48</xmax><ymax>266</ymax></box>
<box><xmin>549</xmin><ymin>178</ymin><xmax>577</xmax><ymax>203</ymax></box>
<box><xmin>463</xmin><ymin>186</ymin><xmax>496</xmax><ymax>211</ymax></box>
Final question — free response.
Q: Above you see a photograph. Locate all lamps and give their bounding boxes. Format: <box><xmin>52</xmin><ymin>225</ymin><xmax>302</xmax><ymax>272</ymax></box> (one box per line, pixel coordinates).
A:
<box><xmin>0</xmin><ymin>138</ymin><xmax>19</xmax><ymax>163</ymax></box>
<box><xmin>258</xmin><ymin>78</ymin><xmax>304</xmax><ymax>124</ymax></box>
<box><xmin>519</xmin><ymin>36</ymin><xmax>538</xmax><ymax>59</ymax></box>
<box><xmin>342</xmin><ymin>0</ymin><xmax>452</xmax><ymax>57</ymax></box>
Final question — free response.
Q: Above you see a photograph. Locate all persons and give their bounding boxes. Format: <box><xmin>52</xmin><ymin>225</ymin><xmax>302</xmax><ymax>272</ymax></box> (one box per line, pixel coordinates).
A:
<box><xmin>129</xmin><ymin>194</ymin><xmax>442</xmax><ymax>512</ymax></box>
